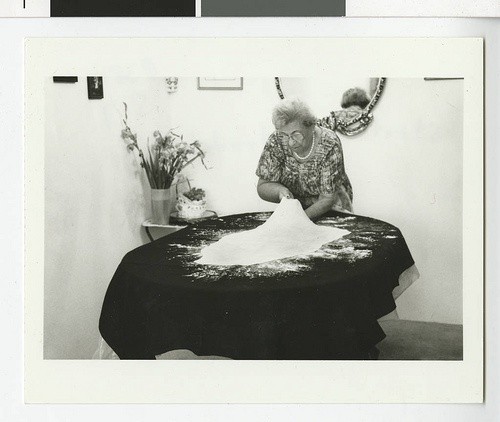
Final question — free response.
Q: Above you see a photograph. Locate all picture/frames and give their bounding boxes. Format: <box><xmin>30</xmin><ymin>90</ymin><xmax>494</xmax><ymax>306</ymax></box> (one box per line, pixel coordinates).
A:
<box><xmin>87</xmin><ymin>77</ymin><xmax>103</xmax><ymax>99</ymax></box>
<box><xmin>197</xmin><ymin>78</ymin><xmax>244</xmax><ymax>90</ymax></box>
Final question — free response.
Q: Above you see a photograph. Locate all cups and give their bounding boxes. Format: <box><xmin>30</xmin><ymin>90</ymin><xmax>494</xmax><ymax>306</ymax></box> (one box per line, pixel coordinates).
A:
<box><xmin>147</xmin><ymin>187</ymin><xmax>171</xmax><ymax>224</ymax></box>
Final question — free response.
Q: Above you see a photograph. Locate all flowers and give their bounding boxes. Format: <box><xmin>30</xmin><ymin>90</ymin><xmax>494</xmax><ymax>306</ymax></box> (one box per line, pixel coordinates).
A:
<box><xmin>120</xmin><ymin>103</ymin><xmax>210</xmax><ymax>188</ymax></box>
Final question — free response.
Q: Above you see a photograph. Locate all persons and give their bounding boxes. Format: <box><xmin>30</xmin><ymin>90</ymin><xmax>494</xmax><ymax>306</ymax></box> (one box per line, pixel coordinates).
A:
<box><xmin>255</xmin><ymin>98</ymin><xmax>354</xmax><ymax>219</ymax></box>
<box><xmin>341</xmin><ymin>87</ymin><xmax>371</xmax><ymax>111</ymax></box>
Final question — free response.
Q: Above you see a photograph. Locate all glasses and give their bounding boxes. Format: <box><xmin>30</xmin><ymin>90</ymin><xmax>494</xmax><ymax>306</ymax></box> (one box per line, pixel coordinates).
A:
<box><xmin>279</xmin><ymin>132</ymin><xmax>303</xmax><ymax>142</ymax></box>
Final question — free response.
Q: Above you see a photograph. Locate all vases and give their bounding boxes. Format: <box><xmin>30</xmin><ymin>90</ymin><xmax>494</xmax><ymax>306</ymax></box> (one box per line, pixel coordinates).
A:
<box><xmin>149</xmin><ymin>189</ymin><xmax>174</xmax><ymax>224</ymax></box>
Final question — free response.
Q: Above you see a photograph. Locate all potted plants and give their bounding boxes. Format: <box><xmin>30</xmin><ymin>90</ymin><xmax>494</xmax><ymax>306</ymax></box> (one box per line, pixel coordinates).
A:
<box><xmin>175</xmin><ymin>185</ymin><xmax>206</xmax><ymax>221</ymax></box>
